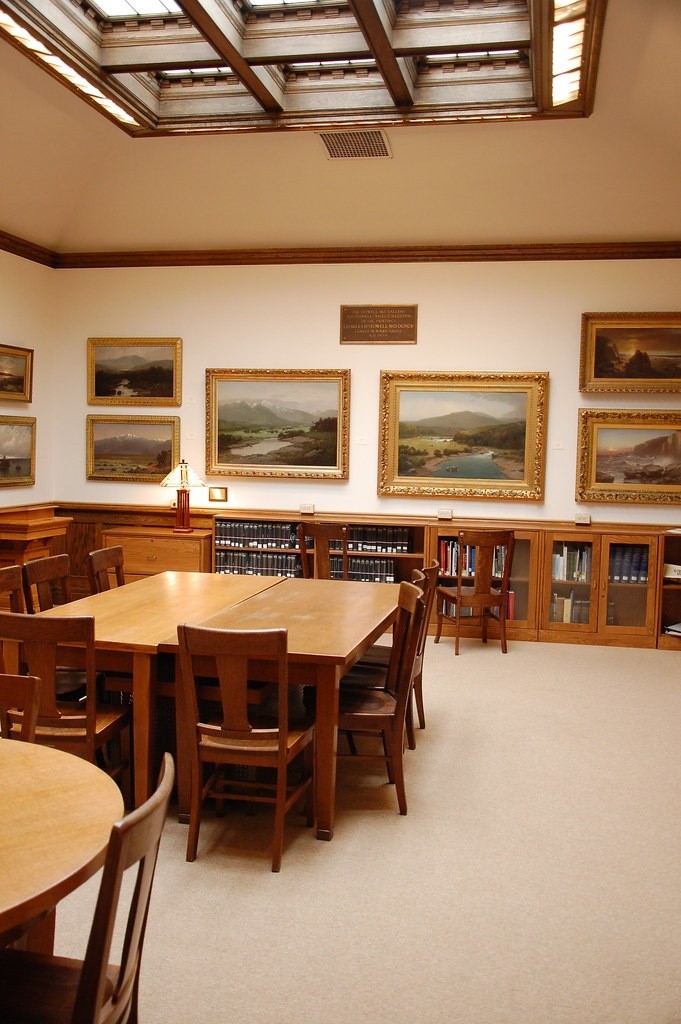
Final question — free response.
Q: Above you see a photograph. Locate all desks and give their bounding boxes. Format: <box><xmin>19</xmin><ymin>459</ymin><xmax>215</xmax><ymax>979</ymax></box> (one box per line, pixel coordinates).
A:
<box><xmin>0</xmin><ymin>571</ymin><xmax>287</xmax><ymax>811</ymax></box>
<box><xmin>0</xmin><ymin>739</ymin><xmax>127</xmax><ymax>955</ymax></box>
<box><xmin>158</xmin><ymin>577</ymin><xmax>400</xmax><ymax>841</ymax></box>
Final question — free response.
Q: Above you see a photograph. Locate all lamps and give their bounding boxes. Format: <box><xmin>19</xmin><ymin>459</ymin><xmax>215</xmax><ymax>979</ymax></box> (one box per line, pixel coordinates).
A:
<box><xmin>0</xmin><ymin>0</ymin><xmax>610</xmax><ymax>140</ymax></box>
<box><xmin>160</xmin><ymin>459</ymin><xmax>207</xmax><ymax>535</ymax></box>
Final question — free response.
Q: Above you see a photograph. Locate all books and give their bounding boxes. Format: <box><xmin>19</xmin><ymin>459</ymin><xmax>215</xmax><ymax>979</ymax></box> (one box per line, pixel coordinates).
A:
<box><xmin>215</xmin><ymin>521</ymin><xmax>314</xmax><ymax>578</ymax></box>
<box><xmin>437</xmin><ymin>539</ymin><xmax>515</xmax><ymax>620</ymax></box>
<box><xmin>608</xmin><ymin>545</ymin><xmax>648</xmax><ymax>583</ymax></box>
<box><xmin>606</xmin><ymin>600</ymin><xmax>614</xmax><ymax>625</ymax></box>
<box><xmin>329</xmin><ymin>526</ymin><xmax>409</xmax><ymax>583</ymax></box>
<box><xmin>551</xmin><ymin>542</ymin><xmax>591</xmax><ymax>624</ymax></box>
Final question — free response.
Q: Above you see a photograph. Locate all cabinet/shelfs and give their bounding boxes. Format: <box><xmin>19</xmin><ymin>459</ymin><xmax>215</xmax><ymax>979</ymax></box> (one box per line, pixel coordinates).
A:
<box><xmin>0</xmin><ymin>500</ymin><xmax>681</xmax><ymax>651</ymax></box>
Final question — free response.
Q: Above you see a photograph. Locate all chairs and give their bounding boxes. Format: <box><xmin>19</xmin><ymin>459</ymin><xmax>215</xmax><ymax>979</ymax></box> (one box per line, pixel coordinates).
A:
<box><xmin>0</xmin><ymin>525</ymin><xmax>515</xmax><ymax>1023</ymax></box>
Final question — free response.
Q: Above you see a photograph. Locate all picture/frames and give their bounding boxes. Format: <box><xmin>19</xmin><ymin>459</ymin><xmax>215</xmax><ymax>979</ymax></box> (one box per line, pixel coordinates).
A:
<box><xmin>205</xmin><ymin>368</ymin><xmax>351</xmax><ymax>480</ymax></box>
<box><xmin>0</xmin><ymin>415</ymin><xmax>37</xmax><ymax>487</ymax></box>
<box><xmin>377</xmin><ymin>370</ymin><xmax>549</xmax><ymax>504</ymax></box>
<box><xmin>579</xmin><ymin>311</ymin><xmax>681</xmax><ymax>391</ymax></box>
<box><xmin>86</xmin><ymin>415</ymin><xmax>180</xmax><ymax>483</ymax></box>
<box><xmin>87</xmin><ymin>337</ymin><xmax>182</xmax><ymax>406</ymax></box>
<box><xmin>0</xmin><ymin>344</ymin><xmax>34</xmax><ymax>403</ymax></box>
<box><xmin>575</xmin><ymin>407</ymin><xmax>681</xmax><ymax>507</ymax></box>
